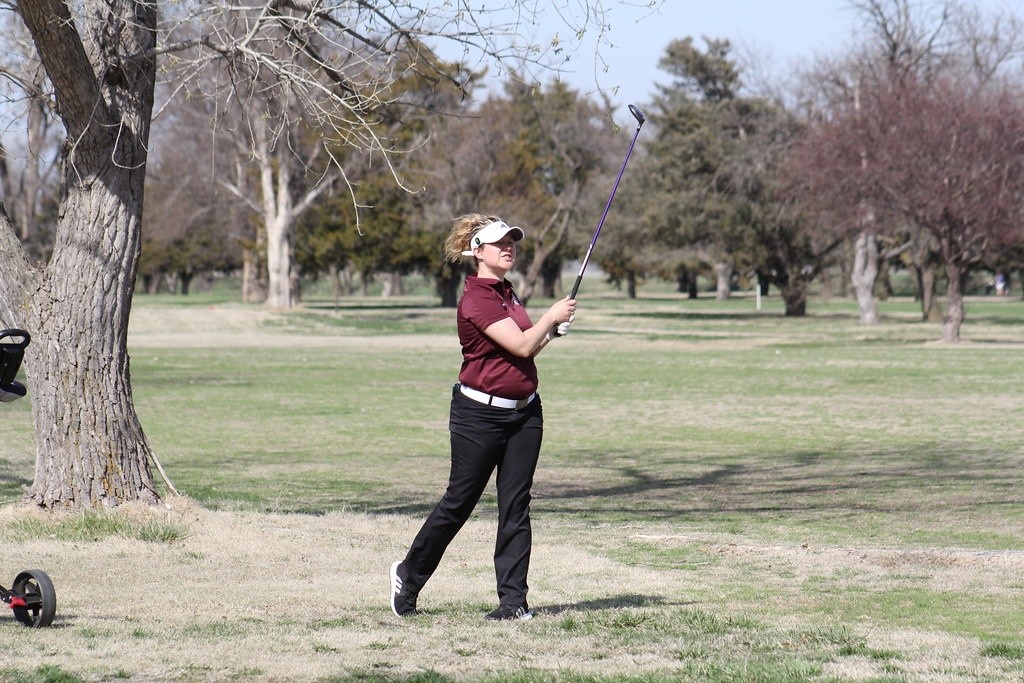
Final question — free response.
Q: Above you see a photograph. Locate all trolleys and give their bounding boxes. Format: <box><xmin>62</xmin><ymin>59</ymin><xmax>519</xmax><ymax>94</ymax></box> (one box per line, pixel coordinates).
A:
<box><xmin>0</xmin><ymin>327</ymin><xmax>57</xmax><ymax>631</ymax></box>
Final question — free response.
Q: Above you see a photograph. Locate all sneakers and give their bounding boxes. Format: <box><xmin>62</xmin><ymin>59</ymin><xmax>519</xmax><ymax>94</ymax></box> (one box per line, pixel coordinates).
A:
<box><xmin>482</xmin><ymin>604</ymin><xmax>533</xmax><ymax>623</ymax></box>
<box><xmin>390</xmin><ymin>561</ymin><xmax>418</xmax><ymax>618</ymax></box>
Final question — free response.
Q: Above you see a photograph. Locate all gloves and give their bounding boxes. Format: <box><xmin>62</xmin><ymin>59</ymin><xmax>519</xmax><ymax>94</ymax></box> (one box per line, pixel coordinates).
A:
<box><xmin>546</xmin><ymin>315</ymin><xmax>575</xmax><ymax>341</ymax></box>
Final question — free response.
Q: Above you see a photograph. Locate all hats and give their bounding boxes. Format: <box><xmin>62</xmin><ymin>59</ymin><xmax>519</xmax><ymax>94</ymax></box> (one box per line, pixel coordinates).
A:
<box><xmin>462</xmin><ymin>220</ymin><xmax>524</xmax><ymax>256</ymax></box>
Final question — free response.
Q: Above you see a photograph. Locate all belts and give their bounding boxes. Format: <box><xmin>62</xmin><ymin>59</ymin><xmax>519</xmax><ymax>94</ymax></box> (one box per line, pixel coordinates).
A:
<box><xmin>460</xmin><ymin>384</ymin><xmax>538</xmax><ymax>409</ymax></box>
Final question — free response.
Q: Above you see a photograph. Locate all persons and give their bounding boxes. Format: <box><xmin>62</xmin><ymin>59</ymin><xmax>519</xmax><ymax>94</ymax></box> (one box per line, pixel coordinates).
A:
<box><xmin>389</xmin><ymin>211</ymin><xmax>577</xmax><ymax>624</ymax></box>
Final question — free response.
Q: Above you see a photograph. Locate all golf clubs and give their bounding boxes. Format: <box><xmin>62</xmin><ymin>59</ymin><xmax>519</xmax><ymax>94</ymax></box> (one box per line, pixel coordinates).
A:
<box><xmin>554</xmin><ymin>103</ymin><xmax>646</xmax><ymax>338</ymax></box>
